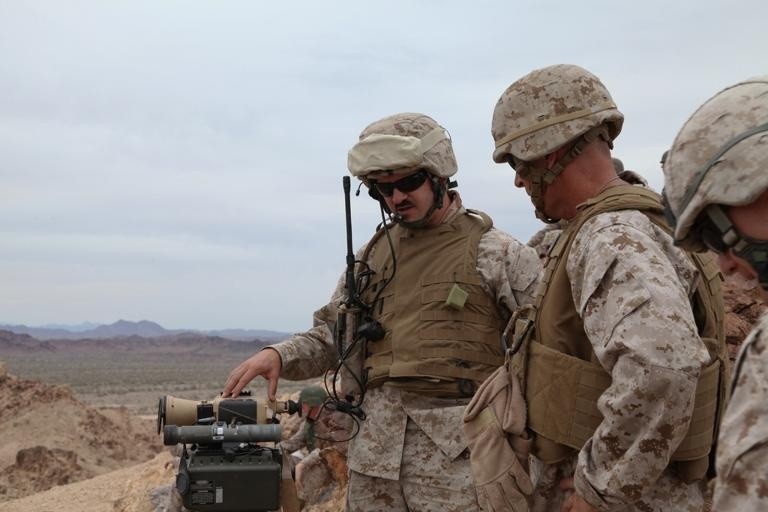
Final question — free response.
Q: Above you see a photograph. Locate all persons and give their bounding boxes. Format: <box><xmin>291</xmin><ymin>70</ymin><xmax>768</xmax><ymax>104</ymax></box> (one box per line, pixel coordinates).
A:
<box><xmin>653</xmin><ymin>71</ymin><xmax>767</xmax><ymax>510</ymax></box>
<box><xmin>219</xmin><ymin>110</ymin><xmax>545</xmax><ymax>512</ymax></box>
<box><xmin>276</xmin><ymin>384</ymin><xmax>339</xmax><ymax>512</ymax></box>
<box><xmin>484</xmin><ymin>60</ymin><xmax>728</xmax><ymax>509</ymax></box>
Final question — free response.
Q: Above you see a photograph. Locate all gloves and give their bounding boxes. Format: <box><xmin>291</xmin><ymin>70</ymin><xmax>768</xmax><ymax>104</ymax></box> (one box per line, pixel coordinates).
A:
<box><xmin>457</xmin><ymin>406</ymin><xmax>535</xmax><ymax>511</ymax></box>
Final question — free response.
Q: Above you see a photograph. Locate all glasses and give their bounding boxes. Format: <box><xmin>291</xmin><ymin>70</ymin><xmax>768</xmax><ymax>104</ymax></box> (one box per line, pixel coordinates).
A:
<box><xmin>365</xmin><ymin>170</ymin><xmax>428</xmax><ymax>197</ymax></box>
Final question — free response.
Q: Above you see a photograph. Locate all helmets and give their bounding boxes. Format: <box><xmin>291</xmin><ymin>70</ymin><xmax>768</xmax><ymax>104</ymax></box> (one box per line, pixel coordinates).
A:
<box><xmin>659</xmin><ymin>73</ymin><xmax>767</xmax><ymax>253</ymax></box>
<box><xmin>347</xmin><ymin>112</ymin><xmax>459</xmax><ymax>184</ymax></box>
<box><xmin>491</xmin><ymin>63</ymin><xmax>626</xmax><ymax>164</ymax></box>
<box><xmin>296</xmin><ymin>386</ymin><xmax>328</xmax><ymax>408</ymax></box>
